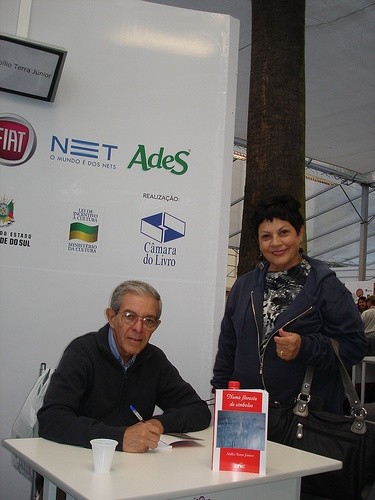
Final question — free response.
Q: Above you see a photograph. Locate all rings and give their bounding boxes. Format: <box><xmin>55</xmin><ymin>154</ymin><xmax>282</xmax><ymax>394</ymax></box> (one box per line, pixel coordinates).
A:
<box><xmin>281</xmin><ymin>351</ymin><xmax>284</xmax><ymax>356</ymax></box>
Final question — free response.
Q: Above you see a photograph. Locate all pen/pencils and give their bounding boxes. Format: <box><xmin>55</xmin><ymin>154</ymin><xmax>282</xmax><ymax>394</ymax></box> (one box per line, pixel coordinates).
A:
<box><xmin>130</xmin><ymin>405</ymin><xmax>147</xmax><ymax>423</ymax></box>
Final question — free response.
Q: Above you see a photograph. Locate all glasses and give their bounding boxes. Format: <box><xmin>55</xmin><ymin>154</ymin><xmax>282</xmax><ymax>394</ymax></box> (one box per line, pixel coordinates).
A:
<box><xmin>114</xmin><ymin>310</ymin><xmax>159</xmax><ymax>331</ymax></box>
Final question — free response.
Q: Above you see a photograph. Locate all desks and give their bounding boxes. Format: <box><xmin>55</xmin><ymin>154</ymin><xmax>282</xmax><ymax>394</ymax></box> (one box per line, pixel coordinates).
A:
<box><xmin>1</xmin><ymin>425</ymin><xmax>342</xmax><ymax>500</ymax></box>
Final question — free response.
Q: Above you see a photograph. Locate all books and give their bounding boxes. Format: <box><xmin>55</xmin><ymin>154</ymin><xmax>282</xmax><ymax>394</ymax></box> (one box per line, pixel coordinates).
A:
<box><xmin>210</xmin><ymin>387</ymin><xmax>269</xmax><ymax>478</ymax></box>
<box><xmin>147</xmin><ymin>431</ymin><xmax>204</xmax><ymax>453</ymax></box>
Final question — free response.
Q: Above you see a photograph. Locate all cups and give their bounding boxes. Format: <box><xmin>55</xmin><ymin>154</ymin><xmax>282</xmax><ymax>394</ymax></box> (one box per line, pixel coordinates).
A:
<box><xmin>89</xmin><ymin>439</ymin><xmax>118</xmax><ymax>473</ymax></box>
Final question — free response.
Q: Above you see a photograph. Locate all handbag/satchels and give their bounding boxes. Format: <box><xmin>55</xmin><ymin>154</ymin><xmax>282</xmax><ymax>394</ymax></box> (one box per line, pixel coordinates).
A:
<box><xmin>283</xmin><ymin>339</ymin><xmax>375</xmax><ymax>500</ymax></box>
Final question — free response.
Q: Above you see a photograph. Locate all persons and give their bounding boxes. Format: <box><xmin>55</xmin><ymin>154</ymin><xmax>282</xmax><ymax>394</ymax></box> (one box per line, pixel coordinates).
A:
<box><xmin>34</xmin><ymin>280</ymin><xmax>211</xmax><ymax>500</ymax></box>
<box><xmin>360</xmin><ymin>295</ymin><xmax>375</xmax><ymax>356</ymax></box>
<box><xmin>358</xmin><ymin>297</ymin><xmax>369</xmax><ymax>315</ymax></box>
<box><xmin>353</xmin><ymin>288</ymin><xmax>363</xmax><ymax>304</ymax></box>
<box><xmin>210</xmin><ymin>201</ymin><xmax>370</xmax><ymax>416</ymax></box>
